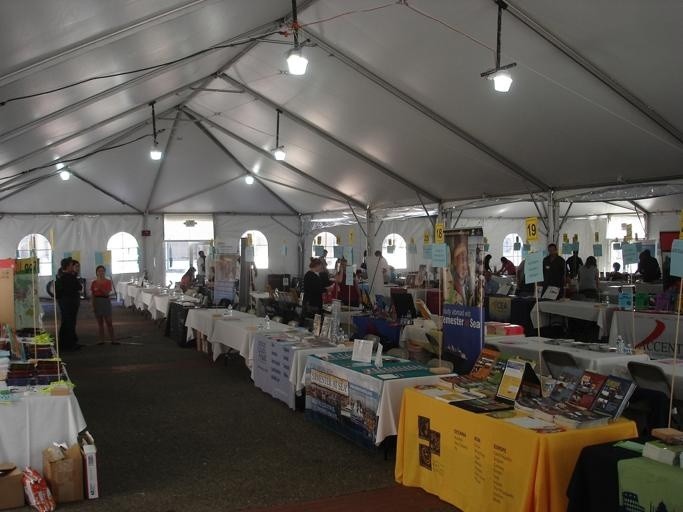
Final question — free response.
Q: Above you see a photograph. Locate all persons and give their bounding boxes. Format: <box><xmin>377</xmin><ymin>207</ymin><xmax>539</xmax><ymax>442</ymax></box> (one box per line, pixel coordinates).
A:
<box><xmin>91</xmin><ymin>265</ymin><xmax>122</xmax><ymax>346</ymax></box>
<box><xmin>301</xmin><ymin>250</ymin><xmax>396</xmax><ymax>324</ymax></box>
<box><xmin>179</xmin><ymin>266</ymin><xmax>196</xmax><ymax>293</ymax></box>
<box><xmin>196</xmin><ymin>251</ymin><xmax>206</xmax><ymax>283</ymax></box>
<box><xmin>475</xmin><ymin>243</ymin><xmax>660</xmax><ymax>300</ymax></box>
<box><xmin>53</xmin><ymin>256</ymin><xmax>81</xmax><ymax>349</ymax></box>
<box><xmin>70</xmin><ymin>260</ymin><xmax>82</xmax><ymax>345</ymax></box>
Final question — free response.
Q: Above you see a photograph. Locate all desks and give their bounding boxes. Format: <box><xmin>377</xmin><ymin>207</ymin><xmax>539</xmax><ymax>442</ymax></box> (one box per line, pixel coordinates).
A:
<box><xmin>118</xmin><ymin>276</ymin><xmax>257</xmax><ymax>385</ymax></box>
<box><xmin>0</xmin><ymin>325</ymin><xmax>88</xmax><ymax>510</ymax></box>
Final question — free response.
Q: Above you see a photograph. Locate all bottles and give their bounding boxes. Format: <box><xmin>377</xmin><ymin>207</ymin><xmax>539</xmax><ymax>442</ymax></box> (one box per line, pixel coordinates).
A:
<box><xmin>227</xmin><ymin>304</ymin><xmax>232</xmax><ymax>316</ymax></box>
<box><xmin>265</xmin><ymin>314</ymin><xmax>270</xmax><ymax>330</ymax></box>
<box><xmin>128</xmin><ymin>276</ymin><xmax>185</xmax><ymax>302</ymax></box>
<box><xmin>615</xmin><ymin>335</ymin><xmax>624</xmax><ymax>353</ymax></box>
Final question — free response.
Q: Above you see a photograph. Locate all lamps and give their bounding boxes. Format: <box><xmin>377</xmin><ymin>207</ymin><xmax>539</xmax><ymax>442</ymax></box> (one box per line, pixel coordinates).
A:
<box><xmin>283</xmin><ymin>0</ymin><xmax>310</xmax><ymax>79</ymax></box>
<box><xmin>147</xmin><ymin>101</ymin><xmax>167</xmax><ymax>163</ymax></box>
<box><xmin>479</xmin><ymin>0</ymin><xmax>519</xmax><ymax>95</ymax></box>
<box><xmin>271</xmin><ymin>108</ymin><xmax>289</xmax><ymax>162</ymax></box>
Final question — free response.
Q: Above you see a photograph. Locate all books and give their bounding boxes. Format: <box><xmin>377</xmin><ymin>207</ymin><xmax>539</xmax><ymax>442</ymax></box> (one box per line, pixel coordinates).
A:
<box><xmin>483</xmin><ymin>321</ymin><xmax>524</xmax><ymax>335</ymax></box>
<box><xmin>642</xmin><ymin>428</ymin><xmax>683</xmax><ymax>465</ymax></box>
<box><xmin>0</xmin><ymin>335</ymin><xmax>68</xmax><ymax>388</ymax></box>
<box><xmin>411</xmin><ymin>346</ymin><xmax>638</xmax><ymax>433</ymax></box>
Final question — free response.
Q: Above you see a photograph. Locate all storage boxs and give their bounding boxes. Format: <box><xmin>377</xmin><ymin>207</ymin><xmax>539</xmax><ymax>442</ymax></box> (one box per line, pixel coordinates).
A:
<box><xmin>0</xmin><ymin>463</ymin><xmax>25</xmax><ymax>512</ymax></box>
<box><xmin>42</xmin><ymin>443</ymin><xmax>84</xmax><ymax>503</ymax></box>
